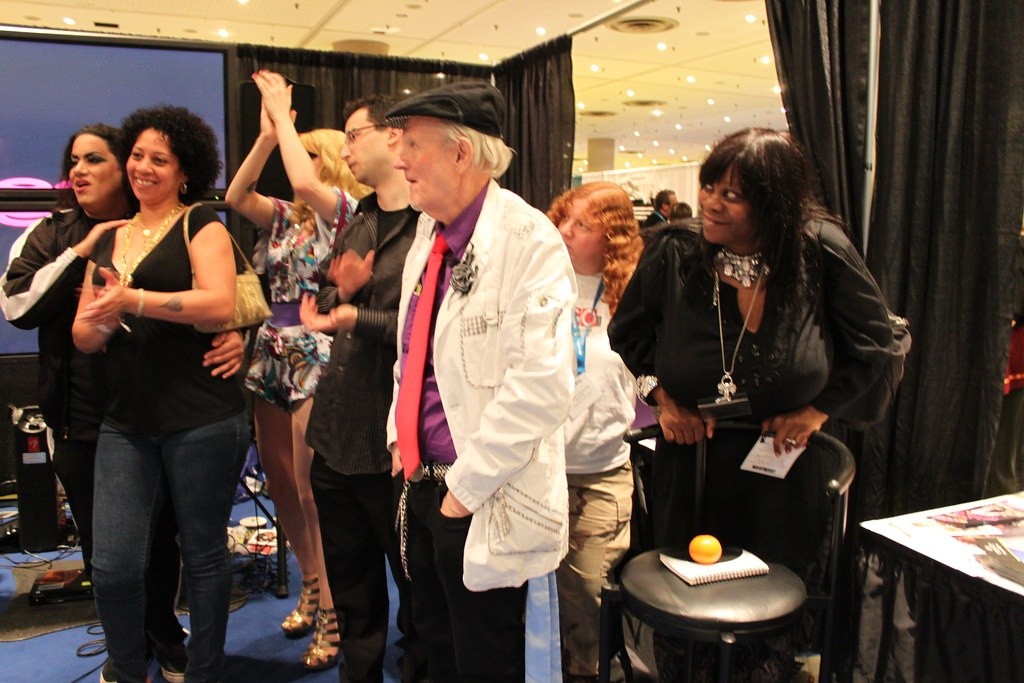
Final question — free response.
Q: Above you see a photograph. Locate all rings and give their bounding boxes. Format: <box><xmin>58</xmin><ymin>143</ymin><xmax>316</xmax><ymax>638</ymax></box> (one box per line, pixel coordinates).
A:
<box><xmin>786</xmin><ymin>438</ymin><xmax>796</xmax><ymax>445</ymax></box>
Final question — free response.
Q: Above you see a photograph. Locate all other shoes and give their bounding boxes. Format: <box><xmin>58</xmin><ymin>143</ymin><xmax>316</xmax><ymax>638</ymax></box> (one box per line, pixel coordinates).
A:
<box><xmin>160</xmin><ymin>655</ymin><xmax>188</xmax><ymax>682</ymax></box>
<box><xmin>100</xmin><ymin>661</ymin><xmax>117</xmax><ymax>682</ymax></box>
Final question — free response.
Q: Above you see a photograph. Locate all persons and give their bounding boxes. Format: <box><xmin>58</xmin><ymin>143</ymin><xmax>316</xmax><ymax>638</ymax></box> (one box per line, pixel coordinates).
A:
<box><xmin>545</xmin><ymin>181</ymin><xmax>645</xmax><ymax>683</ymax></box>
<box><xmin>0</xmin><ymin>121</ymin><xmax>244</xmax><ymax>683</ymax></box>
<box><xmin>384</xmin><ymin>80</ymin><xmax>577</xmax><ymax>683</ymax></box>
<box><xmin>643</xmin><ymin>190</ymin><xmax>692</xmax><ymax>228</ymax></box>
<box><xmin>75</xmin><ymin>105</ymin><xmax>247</xmax><ymax>683</ymax></box>
<box><xmin>226</xmin><ymin>69</ymin><xmax>375</xmax><ymax>670</ymax></box>
<box><xmin>607</xmin><ymin>128</ymin><xmax>893</xmax><ymax>457</ymax></box>
<box><xmin>298</xmin><ymin>93</ymin><xmax>423</xmax><ymax>683</ymax></box>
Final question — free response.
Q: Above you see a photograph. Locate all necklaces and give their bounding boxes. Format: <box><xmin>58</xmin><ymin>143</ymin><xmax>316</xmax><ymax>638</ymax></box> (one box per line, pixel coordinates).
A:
<box><xmin>120</xmin><ymin>203</ymin><xmax>184</xmax><ymax>287</ymax></box>
<box><xmin>712</xmin><ymin>246</ymin><xmax>769</xmax><ymax>403</ymax></box>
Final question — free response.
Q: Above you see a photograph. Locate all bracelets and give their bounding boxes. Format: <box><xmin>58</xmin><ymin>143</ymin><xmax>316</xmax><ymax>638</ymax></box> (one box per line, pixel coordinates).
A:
<box><xmin>636</xmin><ymin>375</ymin><xmax>659</xmax><ymax>407</ymax></box>
<box><xmin>136</xmin><ymin>288</ymin><xmax>145</xmax><ymax>318</ymax></box>
<box><xmin>96</xmin><ymin>323</ymin><xmax>112</xmax><ymax>336</ymax></box>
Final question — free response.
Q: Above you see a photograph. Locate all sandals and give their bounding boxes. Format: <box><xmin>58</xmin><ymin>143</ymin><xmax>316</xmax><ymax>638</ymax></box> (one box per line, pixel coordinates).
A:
<box><xmin>300</xmin><ymin>607</ymin><xmax>340</xmax><ymax>670</ymax></box>
<box><xmin>282</xmin><ymin>577</ymin><xmax>320</xmax><ymax>634</ymax></box>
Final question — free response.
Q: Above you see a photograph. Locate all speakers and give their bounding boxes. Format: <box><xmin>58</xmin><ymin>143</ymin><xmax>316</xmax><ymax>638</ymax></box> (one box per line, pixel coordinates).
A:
<box><xmin>240</xmin><ymin>76</ymin><xmax>317</xmax><ymax>177</ymax></box>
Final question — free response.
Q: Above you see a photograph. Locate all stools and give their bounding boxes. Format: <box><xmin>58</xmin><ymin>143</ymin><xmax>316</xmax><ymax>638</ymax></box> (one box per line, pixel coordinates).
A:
<box><xmin>595</xmin><ymin>411</ymin><xmax>861</xmax><ymax>683</ymax></box>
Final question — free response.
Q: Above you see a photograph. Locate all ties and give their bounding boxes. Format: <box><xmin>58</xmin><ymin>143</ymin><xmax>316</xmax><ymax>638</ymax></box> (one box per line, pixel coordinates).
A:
<box><xmin>398</xmin><ymin>232</ymin><xmax>448</xmax><ymax>482</ymax></box>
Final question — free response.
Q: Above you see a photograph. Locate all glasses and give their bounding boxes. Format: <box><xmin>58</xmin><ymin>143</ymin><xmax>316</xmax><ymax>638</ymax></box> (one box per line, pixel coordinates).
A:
<box><xmin>347</xmin><ymin>125</ymin><xmax>373</xmax><ymax>143</ymax></box>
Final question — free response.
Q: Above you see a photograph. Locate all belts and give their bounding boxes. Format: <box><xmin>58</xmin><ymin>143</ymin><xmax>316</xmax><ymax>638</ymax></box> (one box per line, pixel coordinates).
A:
<box><xmin>418</xmin><ymin>462</ymin><xmax>449</xmax><ymax>480</ymax></box>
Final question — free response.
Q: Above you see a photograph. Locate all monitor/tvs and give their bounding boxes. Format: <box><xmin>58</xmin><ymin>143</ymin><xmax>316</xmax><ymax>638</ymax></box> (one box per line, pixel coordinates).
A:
<box><xmin>0</xmin><ymin>201</ymin><xmax>241</xmax><ymax>364</ymax></box>
<box><xmin>0</xmin><ymin>29</ymin><xmax>240</xmax><ymax>200</ymax></box>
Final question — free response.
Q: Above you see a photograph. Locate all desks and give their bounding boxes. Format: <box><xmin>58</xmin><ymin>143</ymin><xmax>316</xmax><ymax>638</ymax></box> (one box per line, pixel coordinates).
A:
<box><xmin>851</xmin><ymin>489</ymin><xmax>1024</xmax><ymax>683</ymax></box>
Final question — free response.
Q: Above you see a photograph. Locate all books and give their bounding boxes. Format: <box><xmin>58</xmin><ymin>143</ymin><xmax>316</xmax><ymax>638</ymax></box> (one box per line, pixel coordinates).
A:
<box><xmin>658</xmin><ymin>548</ymin><xmax>769</xmax><ymax>586</ymax></box>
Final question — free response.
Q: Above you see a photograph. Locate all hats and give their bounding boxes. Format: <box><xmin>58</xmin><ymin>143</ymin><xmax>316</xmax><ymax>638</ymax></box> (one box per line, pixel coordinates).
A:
<box><xmin>384</xmin><ymin>83</ymin><xmax>505</xmax><ymax>137</ymax></box>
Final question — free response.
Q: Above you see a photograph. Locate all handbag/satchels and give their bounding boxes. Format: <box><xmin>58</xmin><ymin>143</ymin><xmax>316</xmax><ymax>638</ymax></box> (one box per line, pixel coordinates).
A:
<box><xmin>837</xmin><ymin>310</ymin><xmax>912</xmax><ymax>431</ymax></box>
<box><xmin>183</xmin><ymin>203</ymin><xmax>275</xmax><ymax>333</ymax></box>
